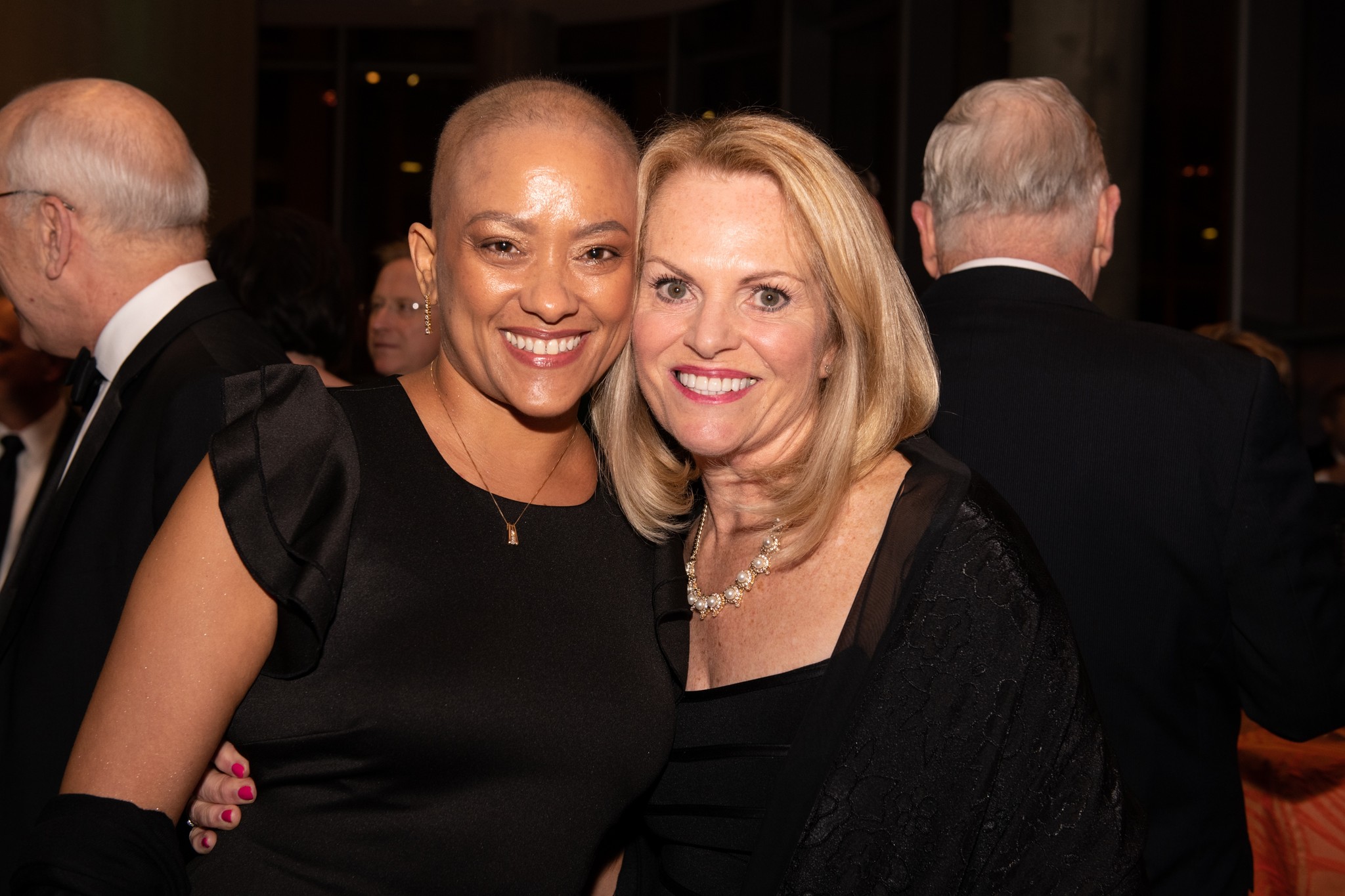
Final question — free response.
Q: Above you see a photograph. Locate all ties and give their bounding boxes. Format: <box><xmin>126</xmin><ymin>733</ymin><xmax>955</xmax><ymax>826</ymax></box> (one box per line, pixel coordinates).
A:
<box><xmin>0</xmin><ymin>434</ymin><xmax>27</xmax><ymax>564</ymax></box>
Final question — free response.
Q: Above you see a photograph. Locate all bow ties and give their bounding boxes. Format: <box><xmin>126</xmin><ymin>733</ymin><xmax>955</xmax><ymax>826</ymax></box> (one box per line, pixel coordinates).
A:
<box><xmin>64</xmin><ymin>347</ymin><xmax>102</xmax><ymax>409</ymax></box>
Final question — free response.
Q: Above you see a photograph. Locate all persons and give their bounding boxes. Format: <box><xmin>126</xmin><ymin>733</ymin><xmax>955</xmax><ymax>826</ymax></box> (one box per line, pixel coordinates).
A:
<box><xmin>3</xmin><ymin>78</ymin><xmax>434</xmax><ymax>896</ymax></box>
<box><xmin>897</xmin><ymin>77</ymin><xmax>1343</xmax><ymax>896</ymax></box>
<box><xmin>590</xmin><ymin>108</ymin><xmax>1123</xmax><ymax>896</ymax></box>
<box><xmin>49</xmin><ymin>76</ymin><xmax>688</xmax><ymax>896</ymax></box>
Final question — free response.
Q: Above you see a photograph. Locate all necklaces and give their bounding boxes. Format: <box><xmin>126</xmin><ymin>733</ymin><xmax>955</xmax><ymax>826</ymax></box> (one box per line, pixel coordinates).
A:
<box><xmin>684</xmin><ymin>497</ymin><xmax>791</xmax><ymax>620</ymax></box>
<box><xmin>430</xmin><ymin>361</ymin><xmax>580</xmax><ymax>546</ymax></box>
